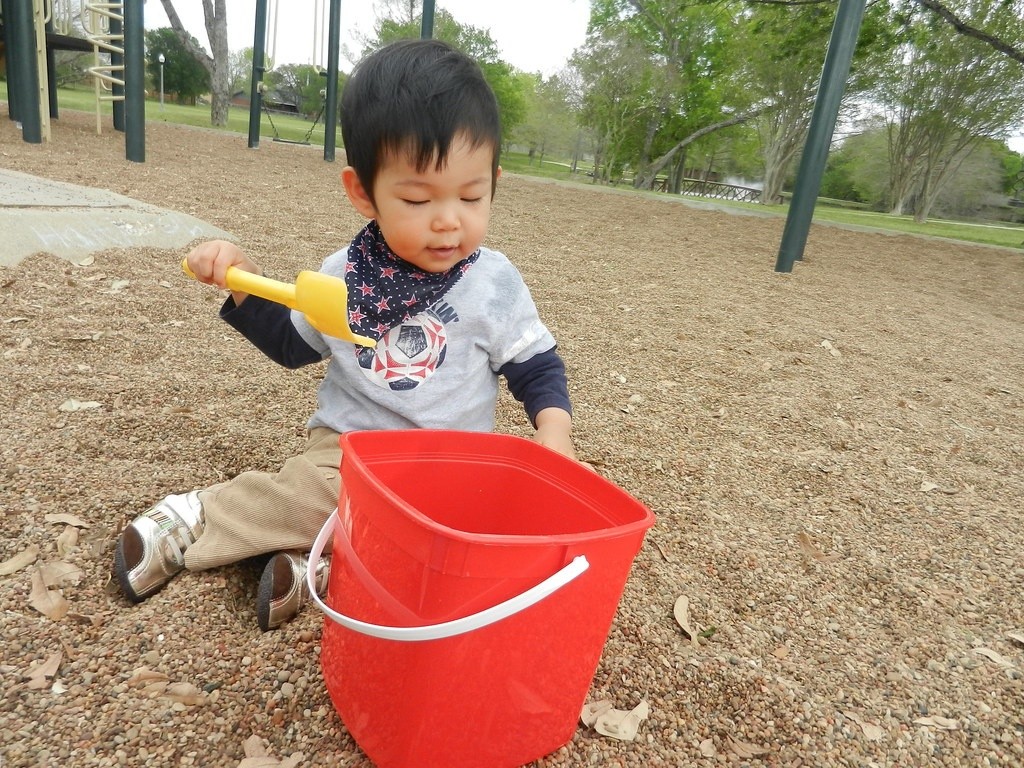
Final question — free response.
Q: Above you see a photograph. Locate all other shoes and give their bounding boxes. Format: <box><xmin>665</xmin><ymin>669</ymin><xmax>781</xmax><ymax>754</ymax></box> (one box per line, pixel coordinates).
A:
<box><xmin>114</xmin><ymin>489</ymin><xmax>205</xmax><ymax>604</ymax></box>
<box><xmin>256</xmin><ymin>549</ymin><xmax>332</xmax><ymax>631</ymax></box>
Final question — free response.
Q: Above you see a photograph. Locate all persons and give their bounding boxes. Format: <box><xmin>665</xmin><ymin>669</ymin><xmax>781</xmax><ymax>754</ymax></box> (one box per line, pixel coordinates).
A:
<box><xmin>115</xmin><ymin>39</ymin><xmax>597</xmax><ymax>633</ymax></box>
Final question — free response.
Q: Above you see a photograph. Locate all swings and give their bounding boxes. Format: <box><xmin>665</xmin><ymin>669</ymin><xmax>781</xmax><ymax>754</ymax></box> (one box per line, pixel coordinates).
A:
<box><xmin>261</xmin><ymin>98</ymin><xmax>326</xmax><ymax>145</ymax></box>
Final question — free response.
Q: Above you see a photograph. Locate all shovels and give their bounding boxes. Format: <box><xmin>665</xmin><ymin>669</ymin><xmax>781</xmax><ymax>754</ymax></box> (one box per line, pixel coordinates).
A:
<box><xmin>181</xmin><ymin>256</ymin><xmax>378</xmax><ymax>348</ymax></box>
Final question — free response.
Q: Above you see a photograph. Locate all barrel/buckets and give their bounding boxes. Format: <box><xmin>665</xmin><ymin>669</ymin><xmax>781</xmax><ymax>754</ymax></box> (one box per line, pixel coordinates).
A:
<box><xmin>306</xmin><ymin>428</ymin><xmax>658</xmax><ymax>768</ymax></box>
